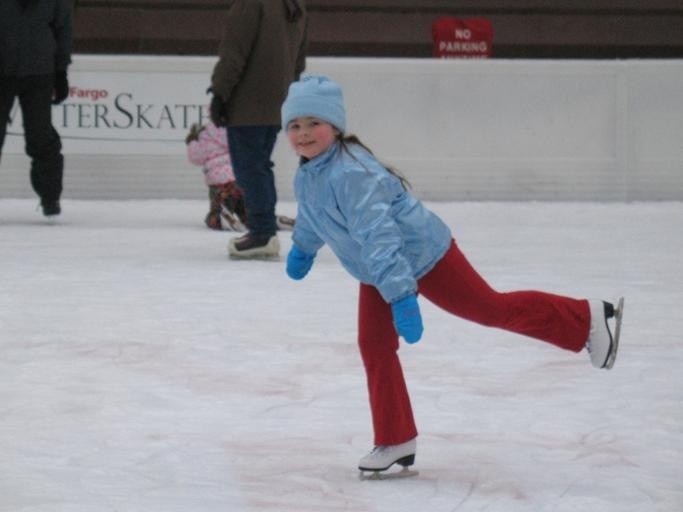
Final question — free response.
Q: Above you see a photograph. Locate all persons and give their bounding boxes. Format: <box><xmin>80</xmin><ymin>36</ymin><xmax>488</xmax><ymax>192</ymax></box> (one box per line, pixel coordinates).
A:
<box><xmin>0</xmin><ymin>0</ymin><xmax>74</xmax><ymax>216</ymax></box>
<box><xmin>186</xmin><ymin>100</ymin><xmax>295</xmax><ymax>233</ymax></box>
<box><xmin>205</xmin><ymin>0</ymin><xmax>311</xmax><ymax>255</ymax></box>
<box><xmin>281</xmin><ymin>74</ymin><xmax>616</xmax><ymax>475</ymax></box>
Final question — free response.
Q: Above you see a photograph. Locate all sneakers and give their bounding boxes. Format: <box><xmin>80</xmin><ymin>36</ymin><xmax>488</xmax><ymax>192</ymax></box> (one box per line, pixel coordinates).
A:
<box><xmin>234</xmin><ymin>232</ymin><xmax>278</xmax><ymax>251</ymax></box>
<box><xmin>358</xmin><ymin>436</ymin><xmax>416</xmax><ymax>470</ymax></box>
<box><xmin>41</xmin><ymin>197</ymin><xmax>60</xmax><ymax>214</ymax></box>
<box><xmin>583</xmin><ymin>298</ymin><xmax>613</xmax><ymax>369</ymax></box>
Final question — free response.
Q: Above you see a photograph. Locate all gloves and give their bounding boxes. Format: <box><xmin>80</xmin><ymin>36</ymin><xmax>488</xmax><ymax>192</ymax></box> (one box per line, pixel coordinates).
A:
<box><xmin>51</xmin><ymin>73</ymin><xmax>68</xmax><ymax>105</ymax></box>
<box><xmin>209</xmin><ymin>97</ymin><xmax>225</xmax><ymax>128</ymax></box>
<box><xmin>390</xmin><ymin>293</ymin><xmax>423</xmax><ymax>343</ymax></box>
<box><xmin>285</xmin><ymin>245</ymin><xmax>314</xmax><ymax>280</ymax></box>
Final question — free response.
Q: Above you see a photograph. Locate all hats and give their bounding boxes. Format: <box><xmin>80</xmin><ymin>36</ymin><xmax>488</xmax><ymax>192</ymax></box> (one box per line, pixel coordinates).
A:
<box><xmin>280</xmin><ymin>74</ymin><xmax>345</xmax><ymax>137</ymax></box>
